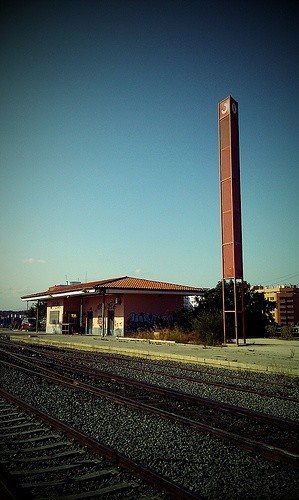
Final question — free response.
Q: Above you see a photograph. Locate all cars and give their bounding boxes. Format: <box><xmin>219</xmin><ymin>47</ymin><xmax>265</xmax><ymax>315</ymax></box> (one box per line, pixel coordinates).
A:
<box><xmin>21</xmin><ymin>317</ymin><xmax>42</xmax><ymax>331</ymax></box>
